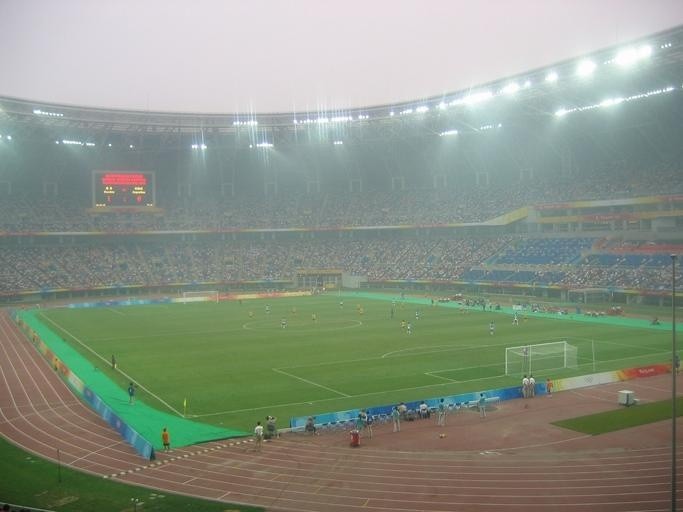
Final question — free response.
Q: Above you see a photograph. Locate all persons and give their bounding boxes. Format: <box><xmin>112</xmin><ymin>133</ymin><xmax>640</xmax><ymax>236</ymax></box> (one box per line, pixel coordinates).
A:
<box><xmin>357</xmin><ymin>304</ymin><xmax>364</xmax><ymax>315</ymax></box>
<box><xmin>391</xmin><ymin>302</ymin><xmax>625</xmax><ymax>336</ymax></box>
<box><xmin>264</xmin><ymin>414</ymin><xmax>281</xmax><ymax>439</ymax></box>
<box><xmin>544</xmin><ymin>377</ymin><xmax>553</xmax><ymax>399</ymax></box>
<box><xmin>264</xmin><ymin>304</ymin><xmax>272</xmax><ymax>313</ymax></box>
<box><xmin>161</xmin><ymin>429</ymin><xmax>171</xmax><ymax>451</ymax></box>
<box><xmin>336</xmin><ymin>301</ymin><xmax>344</xmax><ymax>309</ymax></box>
<box><xmin>521</xmin><ymin>375</ymin><xmax>530</xmax><ymax>398</ymax></box>
<box><xmin>249</xmin><ymin>311</ymin><xmax>256</xmax><ymax>320</ymax></box>
<box><xmin>672</xmin><ymin>356</ymin><xmax>680</xmax><ymax>371</ymax></box>
<box><xmin>528</xmin><ymin>374</ymin><xmax>535</xmax><ymax>397</ymax></box>
<box><xmin>354</xmin><ymin>396</ymin><xmax>449</xmax><ymax>440</ymax></box>
<box><xmin>288</xmin><ymin>307</ymin><xmax>297</xmax><ymax>316</ymax></box>
<box><xmin>0</xmin><ymin>164</ymin><xmax>683</xmax><ymax>302</ymax></box>
<box><xmin>127</xmin><ymin>382</ymin><xmax>137</xmax><ymax>403</ymax></box>
<box><xmin>280</xmin><ymin>316</ymin><xmax>287</xmax><ymax>328</ymax></box>
<box><xmin>312</xmin><ymin>312</ymin><xmax>318</xmax><ymax>322</ymax></box>
<box><xmin>303</xmin><ymin>416</ymin><xmax>319</xmax><ymax>435</ymax></box>
<box><xmin>478</xmin><ymin>392</ymin><xmax>487</xmax><ymax>417</ymax></box>
<box><xmin>521</xmin><ymin>344</ymin><xmax>528</xmax><ymax>367</ymax></box>
<box><xmin>110</xmin><ymin>355</ymin><xmax>120</xmax><ymax>370</ymax></box>
<box><xmin>253</xmin><ymin>421</ymin><xmax>264</xmax><ymax>448</ymax></box>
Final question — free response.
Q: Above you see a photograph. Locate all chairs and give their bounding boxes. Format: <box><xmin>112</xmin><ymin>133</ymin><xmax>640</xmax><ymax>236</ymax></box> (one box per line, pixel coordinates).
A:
<box><xmin>459</xmin><ymin>236</ymin><xmax>682</xmax><ymax>290</ymax></box>
